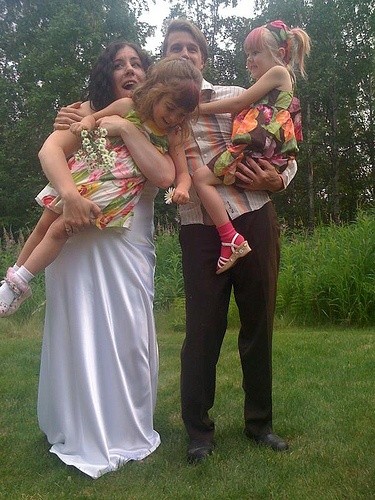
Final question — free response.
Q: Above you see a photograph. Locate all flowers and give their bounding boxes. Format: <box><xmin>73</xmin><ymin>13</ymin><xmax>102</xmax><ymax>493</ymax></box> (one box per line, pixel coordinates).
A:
<box><xmin>74</xmin><ymin>128</ymin><xmax>118</xmax><ymax>174</ymax></box>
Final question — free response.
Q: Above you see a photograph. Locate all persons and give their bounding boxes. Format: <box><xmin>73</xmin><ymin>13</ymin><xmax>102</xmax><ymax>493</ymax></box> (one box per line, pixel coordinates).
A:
<box><xmin>0</xmin><ymin>56</ymin><xmax>202</xmax><ymax>320</ymax></box>
<box><xmin>189</xmin><ymin>20</ymin><xmax>312</xmax><ymax>275</ymax></box>
<box><xmin>53</xmin><ymin>20</ymin><xmax>297</xmax><ymax>465</ymax></box>
<box><xmin>38</xmin><ymin>41</ymin><xmax>176</xmax><ymax>479</ymax></box>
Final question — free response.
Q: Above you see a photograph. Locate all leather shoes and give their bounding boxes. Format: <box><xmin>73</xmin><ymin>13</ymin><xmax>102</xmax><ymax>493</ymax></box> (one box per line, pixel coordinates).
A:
<box><xmin>187</xmin><ymin>440</ymin><xmax>216</xmax><ymax>465</ymax></box>
<box><xmin>244</xmin><ymin>424</ymin><xmax>289</xmax><ymax>452</ymax></box>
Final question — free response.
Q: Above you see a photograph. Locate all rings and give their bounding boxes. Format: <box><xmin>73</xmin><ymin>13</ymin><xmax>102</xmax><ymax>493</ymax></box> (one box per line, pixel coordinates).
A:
<box><xmin>65</xmin><ymin>228</ymin><xmax>72</xmax><ymax>232</ymax></box>
<box><xmin>250</xmin><ymin>180</ymin><xmax>254</xmax><ymax>186</ymax></box>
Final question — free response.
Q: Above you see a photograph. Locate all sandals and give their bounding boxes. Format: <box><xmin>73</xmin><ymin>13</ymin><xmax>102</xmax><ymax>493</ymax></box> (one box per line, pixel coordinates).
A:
<box><xmin>0</xmin><ymin>268</ymin><xmax>32</xmax><ymax>317</ymax></box>
<box><xmin>215</xmin><ymin>240</ymin><xmax>252</xmax><ymax>275</ymax></box>
